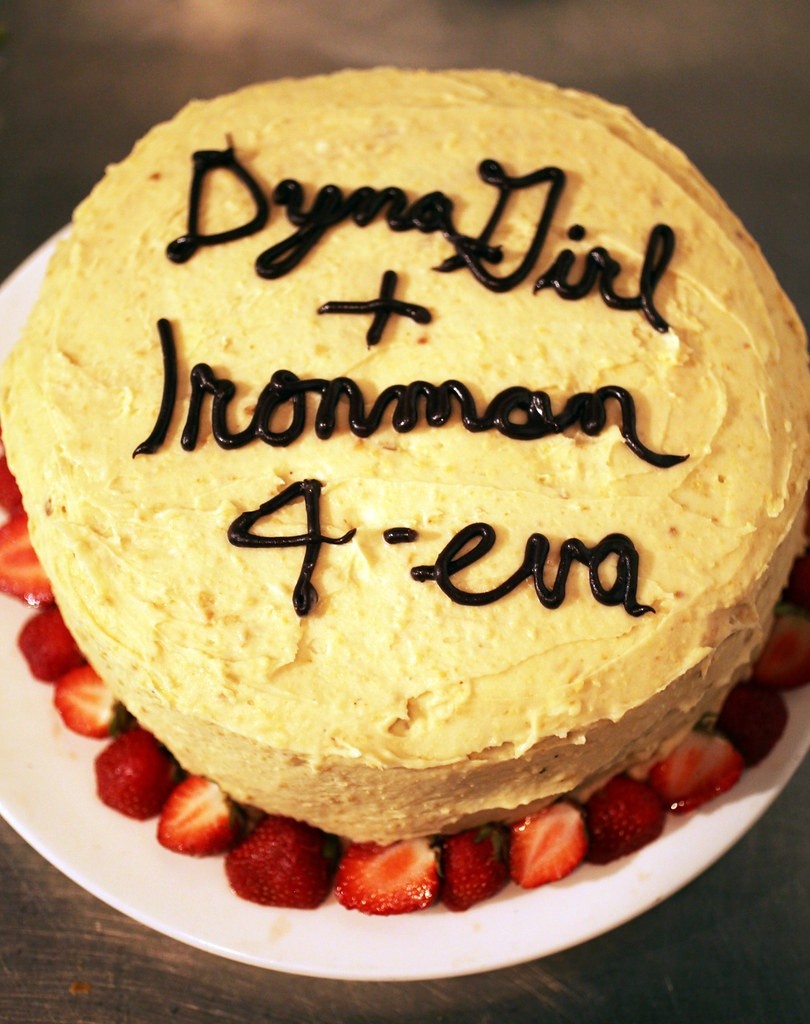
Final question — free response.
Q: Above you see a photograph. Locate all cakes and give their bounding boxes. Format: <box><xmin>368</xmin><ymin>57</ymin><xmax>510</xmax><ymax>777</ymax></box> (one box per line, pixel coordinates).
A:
<box><xmin>0</xmin><ymin>63</ymin><xmax>810</xmax><ymax>842</ymax></box>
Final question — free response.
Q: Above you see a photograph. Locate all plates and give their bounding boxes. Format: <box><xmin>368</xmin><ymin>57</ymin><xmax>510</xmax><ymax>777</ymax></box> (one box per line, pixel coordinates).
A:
<box><xmin>0</xmin><ymin>222</ymin><xmax>810</xmax><ymax>983</ymax></box>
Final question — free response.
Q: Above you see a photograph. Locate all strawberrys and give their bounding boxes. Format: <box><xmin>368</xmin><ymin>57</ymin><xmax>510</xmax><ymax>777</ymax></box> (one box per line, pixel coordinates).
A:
<box><xmin>0</xmin><ymin>474</ymin><xmax>810</xmax><ymax>916</ymax></box>
